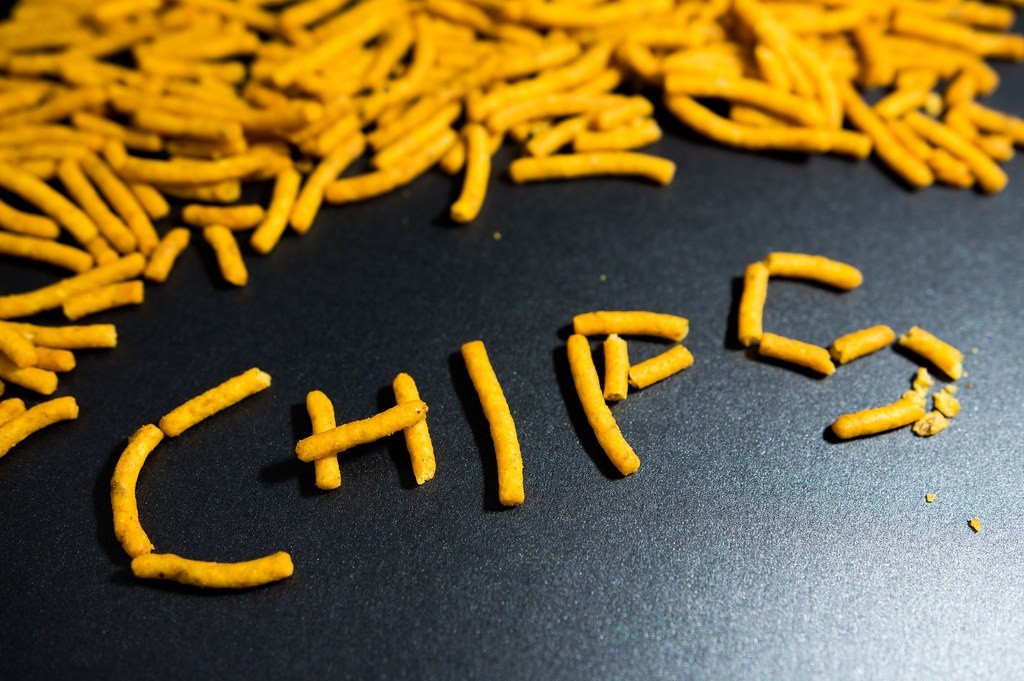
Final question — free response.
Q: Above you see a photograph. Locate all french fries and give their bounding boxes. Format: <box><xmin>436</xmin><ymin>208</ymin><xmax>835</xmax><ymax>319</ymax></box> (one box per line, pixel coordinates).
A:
<box><xmin>0</xmin><ymin>1</ymin><xmax>1024</xmax><ymax>589</ymax></box>
